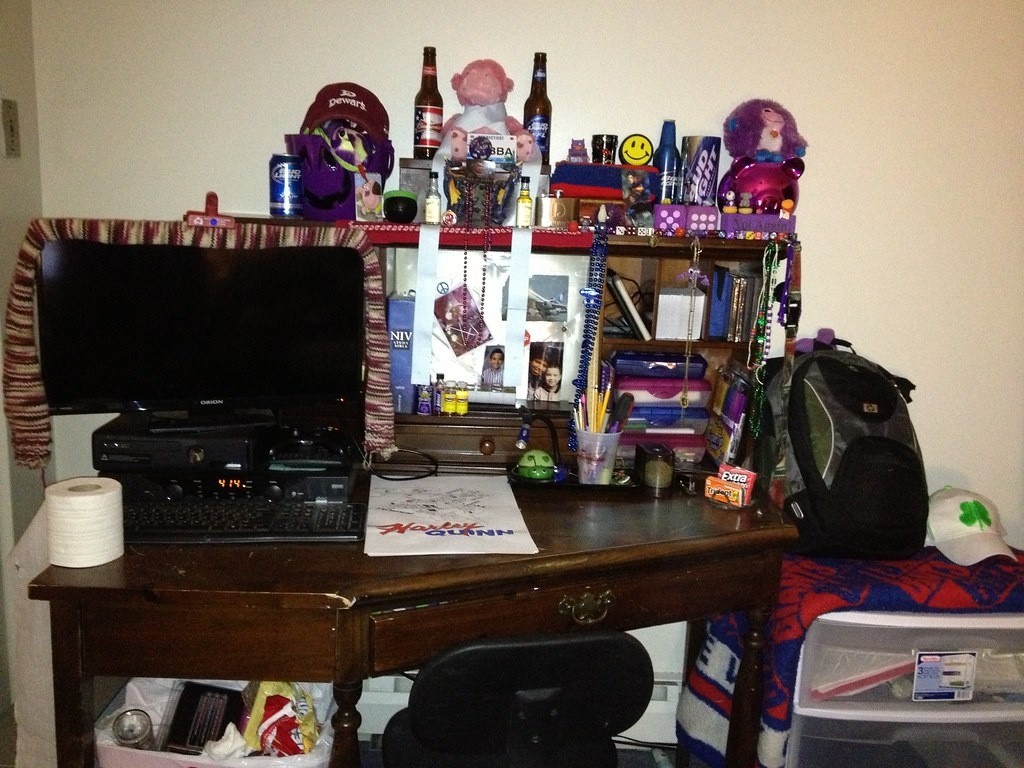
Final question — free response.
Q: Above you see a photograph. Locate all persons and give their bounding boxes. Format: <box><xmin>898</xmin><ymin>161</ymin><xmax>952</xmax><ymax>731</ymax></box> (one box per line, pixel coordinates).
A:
<box><xmin>625</xmin><ymin>171</ymin><xmax>651</xmax><ymax>216</ymax></box>
<box><xmin>528</xmin><ymin>351</ymin><xmax>561</xmax><ymax>402</ymax></box>
<box><xmin>483</xmin><ymin>348</ymin><xmax>504</xmax><ymax>386</ymax></box>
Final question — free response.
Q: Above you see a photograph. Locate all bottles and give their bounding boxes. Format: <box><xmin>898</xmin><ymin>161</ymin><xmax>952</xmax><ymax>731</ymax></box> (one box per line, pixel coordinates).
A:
<box><xmin>114</xmin><ymin>709</ymin><xmax>157</xmax><ymax>751</ymax></box>
<box><xmin>433</xmin><ymin>374</ymin><xmax>445</xmax><ymax>415</ymax></box>
<box><xmin>417</xmin><ymin>385</ymin><xmax>433</xmax><ymax>416</ymax></box>
<box><xmin>444</xmin><ymin>381</ymin><xmax>456</xmax><ymax>415</ymax></box>
<box><xmin>414</xmin><ymin>47</ymin><xmax>443</xmax><ymax>161</ymax></box>
<box><xmin>516</xmin><ymin>177</ymin><xmax>532</xmax><ymax>226</ymax></box>
<box><xmin>652</xmin><ymin>119</ymin><xmax>680</xmax><ymax>206</ymax></box>
<box><xmin>425</xmin><ymin>172</ymin><xmax>441</xmax><ymax>225</ymax></box>
<box><xmin>523</xmin><ymin>52</ymin><xmax>552</xmax><ymax>165</ymax></box>
<box><xmin>456</xmin><ymin>382</ymin><xmax>468</xmax><ymax>416</ymax></box>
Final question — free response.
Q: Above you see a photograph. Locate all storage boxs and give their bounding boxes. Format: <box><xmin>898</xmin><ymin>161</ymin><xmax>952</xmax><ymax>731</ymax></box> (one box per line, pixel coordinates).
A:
<box><xmin>400</xmin><ymin>158</ymin><xmax>551</xmax><ymax>223</ymax></box>
<box><xmin>610</xmin><ymin>349</ymin><xmax>712</xmax><ymax>464</ymax></box>
<box><xmin>387</xmin><ymin>296</ymin><xmax>419</xmax><ymax>414</ymax></box>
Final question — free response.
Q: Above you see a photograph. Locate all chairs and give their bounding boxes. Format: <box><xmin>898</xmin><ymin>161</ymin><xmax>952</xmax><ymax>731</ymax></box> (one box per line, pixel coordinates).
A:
<box><xmin>382</xmin><ymin>633</ymin><xmax>655</xmax><ymax>768</ymax></box>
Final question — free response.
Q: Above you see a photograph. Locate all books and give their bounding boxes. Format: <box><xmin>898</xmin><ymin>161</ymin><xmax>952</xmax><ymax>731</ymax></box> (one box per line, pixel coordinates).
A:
<box><xmin>387</xmin><ymin>290</ymin><xmax>415</xmax><ymax>413</ymax></box>
<box><xmin>709</xmin><ymin>267</ymin><xmax>762</xmax><ymax>343</ymax></box>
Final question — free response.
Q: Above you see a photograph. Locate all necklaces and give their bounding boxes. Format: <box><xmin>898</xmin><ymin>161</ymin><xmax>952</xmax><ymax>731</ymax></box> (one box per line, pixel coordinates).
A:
<box><xmin>681</xmin><ymin>236</ymin><xmax>700</xmax><ymax>407</ymax></box>
<box><xmin>568</xmin><ymin>226</ymin><xmax>606</xmax><ymax>451</ymax></box>
<box><xmin>747</xmin><ymin>241</ymin><xmax>778</xmax><ymax>438</ymax></box>
<box><xmin>461</xmin><ymin>181</ymin><xmax>490</xmax><ymax>347</ymax></box>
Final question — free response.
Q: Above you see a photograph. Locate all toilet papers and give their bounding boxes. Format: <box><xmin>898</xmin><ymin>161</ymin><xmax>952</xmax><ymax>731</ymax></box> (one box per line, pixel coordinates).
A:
<box><xmin>5</xmin><ymin>476</ymin><xmax>124</xmax><ymax>767</ymax></box>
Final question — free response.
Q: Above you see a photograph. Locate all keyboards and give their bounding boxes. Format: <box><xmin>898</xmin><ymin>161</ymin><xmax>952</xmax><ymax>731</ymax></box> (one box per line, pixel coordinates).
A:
<box><xmin>123</xmin><ymin>499</ymin><xmax>368</xmax><ymax>544</ymax></box>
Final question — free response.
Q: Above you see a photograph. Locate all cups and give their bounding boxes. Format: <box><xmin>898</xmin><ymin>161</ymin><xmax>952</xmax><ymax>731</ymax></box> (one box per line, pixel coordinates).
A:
<box><xmin>591</xmin><ymin>135</ymin><xmax>618</xmax><ymax>164</ymax></box>
<box><xmin>574</xmin><ymin>426</ymin><xmax>624</xmax><ymax>485</ymax></box>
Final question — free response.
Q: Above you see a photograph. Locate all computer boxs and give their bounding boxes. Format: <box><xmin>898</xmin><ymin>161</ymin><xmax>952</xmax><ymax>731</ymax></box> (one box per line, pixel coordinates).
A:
<box><xmin>92</xmin><ymin>414</ymin><xmax>265</xmax><ymax>474</ymax></box>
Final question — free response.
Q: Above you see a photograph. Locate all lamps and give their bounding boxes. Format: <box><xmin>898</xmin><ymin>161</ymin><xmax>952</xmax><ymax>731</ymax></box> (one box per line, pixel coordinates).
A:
<box><xmin>516</xmin><ymin>413</ymin><xmax>570</xmax><ymax>482</ymax></box>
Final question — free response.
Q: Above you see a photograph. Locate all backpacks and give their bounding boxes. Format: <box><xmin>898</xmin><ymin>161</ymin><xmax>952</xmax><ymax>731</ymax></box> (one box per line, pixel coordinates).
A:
<box><xmin>760</xmin><ymin>328</ymin><xmax>929</xmax><ymax>556</ymax></box>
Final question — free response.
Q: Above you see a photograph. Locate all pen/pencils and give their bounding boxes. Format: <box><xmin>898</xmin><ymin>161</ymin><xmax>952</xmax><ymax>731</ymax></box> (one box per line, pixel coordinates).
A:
<box><xmin>573</xmin><ymin>381</ymin><xmax>612</xmax><ymax>433</ymax></box>
<box><xmin>589</xmin><ymin>392</ymin><xmax>634</xmax><ymax>480</ymax></box>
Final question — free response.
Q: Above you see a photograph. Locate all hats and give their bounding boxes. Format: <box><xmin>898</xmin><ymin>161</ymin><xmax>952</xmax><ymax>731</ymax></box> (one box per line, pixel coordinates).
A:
<box><xmin>299</xmin><ymin>82</ymin><xmax>390</xmax><ymax>149</ymax></box>
<box><xmin>927</xmin><ymin>490</ymin><xmax>1019</xmax><ymax>567</ymax></box>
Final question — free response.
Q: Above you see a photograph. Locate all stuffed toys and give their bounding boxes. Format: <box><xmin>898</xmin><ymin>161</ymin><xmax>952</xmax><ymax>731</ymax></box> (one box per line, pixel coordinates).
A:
<box><xmin>723</xmin><ymin>98</ymin><xmax>809</xmax><ymax>162</ymax></box>
<box><xmin>440</xmin><ymin>58</ymin><xmax>534</xmax><ymax>161</ymax></box>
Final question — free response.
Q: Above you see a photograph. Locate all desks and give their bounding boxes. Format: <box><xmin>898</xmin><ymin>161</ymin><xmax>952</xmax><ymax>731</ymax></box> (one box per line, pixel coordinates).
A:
<box><xmin>675</xmin><ymin>545</ymin><xmax>1024</xmax><ymax>768</ymax></box>
<box><xmin>26</xmin><ymin>484</ymin><xmax>798</xmax><ymax>768</ymax></box>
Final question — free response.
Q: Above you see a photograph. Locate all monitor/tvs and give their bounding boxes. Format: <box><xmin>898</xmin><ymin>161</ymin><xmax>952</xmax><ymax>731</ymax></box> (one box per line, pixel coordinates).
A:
<box><xmin>39</xmin><ymin>242</ymin><xmax>364</xmax><ymax>433</ymax></box>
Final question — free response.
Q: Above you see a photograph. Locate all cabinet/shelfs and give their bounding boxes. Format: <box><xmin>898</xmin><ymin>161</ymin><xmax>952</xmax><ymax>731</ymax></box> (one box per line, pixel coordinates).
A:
<box><xmin>787</xmin><ymin>613</ymin><xmax>1024</xmax><ymax>768</ymax></box>
<box><xmin>232</xmin><ymin>216</ymin><xmax>788</xmax><ymax>474</ymax></box>
